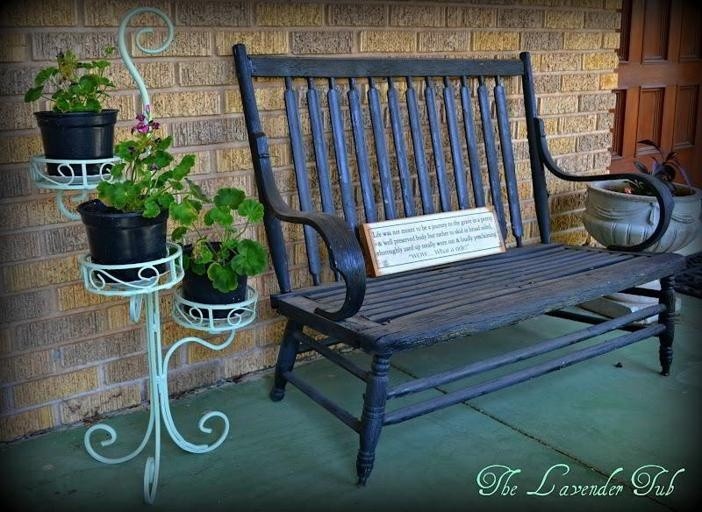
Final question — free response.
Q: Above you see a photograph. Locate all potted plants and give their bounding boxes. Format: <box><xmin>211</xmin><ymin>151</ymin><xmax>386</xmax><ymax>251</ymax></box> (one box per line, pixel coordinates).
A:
<box><xmin>172</xmin><ymin>188</ymin><xmax>270</xmax><ymax>318</ymax></box>
<box><xmin>576</xmin><ymin>140</ymin><xmax>702</xmax><ymax>326</ymax></box>
<box><xmin>77</xmin><ymin>105</ymin><xmax>212</xmax><ymax>283</ymax></box>
<box><xmin>23</xmin><ymin>45</ymin><xmax>120</xmax><ymax>176</ymax></box>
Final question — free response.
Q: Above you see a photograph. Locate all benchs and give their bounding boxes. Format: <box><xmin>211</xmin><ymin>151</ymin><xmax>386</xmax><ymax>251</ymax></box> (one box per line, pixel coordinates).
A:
<box><xmin>231</xmin><ymin>44</ymin><xmax>684</xmax><ymax>486</ymax></box>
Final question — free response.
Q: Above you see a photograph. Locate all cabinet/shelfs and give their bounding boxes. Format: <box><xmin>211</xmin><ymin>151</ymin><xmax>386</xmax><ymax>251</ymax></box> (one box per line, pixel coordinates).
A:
<box><xmin>30</xmin><ymin>6</ymin><xmax>258</xmax><ymax>504</ymax></box>
